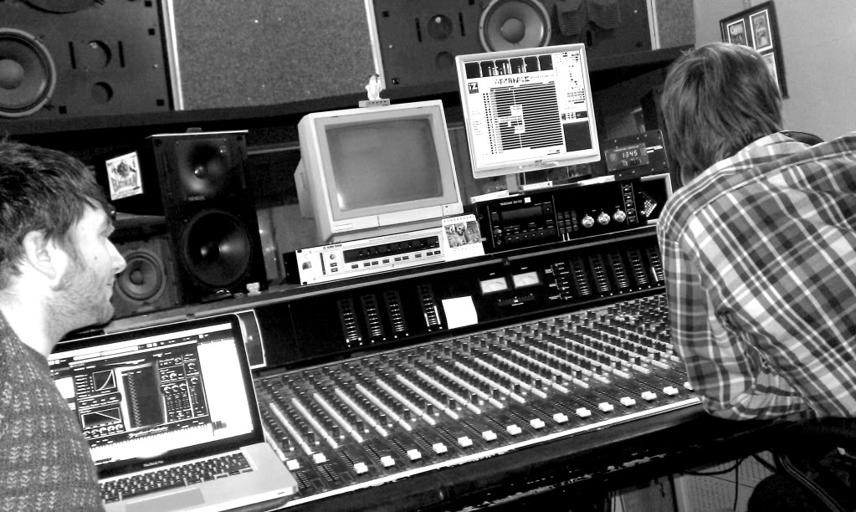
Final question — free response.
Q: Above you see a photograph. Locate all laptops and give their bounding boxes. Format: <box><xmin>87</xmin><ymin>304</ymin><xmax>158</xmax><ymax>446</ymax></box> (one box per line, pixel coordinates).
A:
<box><xmin>47</xmin><ymin>313</ymin><xmax>300</xmax><ymax>512</ymax></box>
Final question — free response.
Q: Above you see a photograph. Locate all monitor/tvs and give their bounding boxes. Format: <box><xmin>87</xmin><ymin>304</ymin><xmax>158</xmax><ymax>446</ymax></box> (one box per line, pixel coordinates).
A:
<box><xmin>454</xmin><ymin>42</ymin><xmax>602</xmax><ymax>195</ymax></box>
<box><xmin>293</xmin><ymin>99</ymin><xmax>464</xmax><ymax>243</ymax></box>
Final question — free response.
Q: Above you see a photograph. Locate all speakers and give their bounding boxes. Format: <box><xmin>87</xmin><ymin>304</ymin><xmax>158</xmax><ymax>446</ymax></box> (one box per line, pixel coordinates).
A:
<box><xmin>370</xmin><ymin>0</ymin><xmax>656</xmax><ymax>99</ymax></box>
<box><xmin>109</xmin><ymin>211</ymin><xmax>183</xmax><ymax>320</ymax></box>
<box><xmin>96</xmin><ymin>130</ymin><xmax>268</xmax><ymax>306</ymax></box>
<box><xmin>0</xmin><ymin>0</ymin><xmax>180</xmax><ymax>137</ymax></box>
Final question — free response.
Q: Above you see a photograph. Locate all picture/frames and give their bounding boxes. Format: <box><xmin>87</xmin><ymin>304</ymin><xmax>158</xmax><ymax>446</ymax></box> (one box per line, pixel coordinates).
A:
<box><xmin>718</xmin><ymin>1</ymin><xmax>788</xmax><ymax>99</ymax></box>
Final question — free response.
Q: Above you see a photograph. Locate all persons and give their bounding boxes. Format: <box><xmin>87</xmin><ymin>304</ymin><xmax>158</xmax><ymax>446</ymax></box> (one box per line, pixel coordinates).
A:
<box><xmin>0</xmin><ymin>140</ymin><xmax>128</xmax><ymax>512</ymax></box>
<box><xmin>656</xmin><ymin>43</ymin><xmax>856</xmax><ymax>512</ymax></box>
